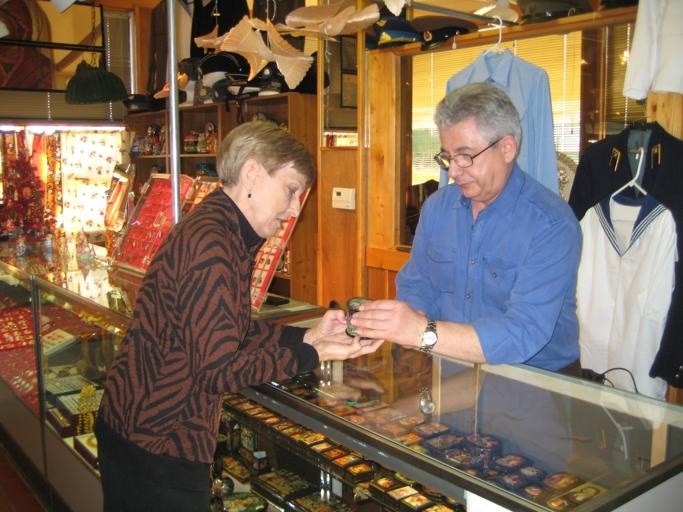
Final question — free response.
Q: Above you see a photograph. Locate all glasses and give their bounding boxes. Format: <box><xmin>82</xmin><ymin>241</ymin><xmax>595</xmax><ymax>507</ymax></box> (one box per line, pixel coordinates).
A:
<box><xmin>433</xmin><ymin>137</ymin><xmax>504</xmax><ymax>170</ymax></box>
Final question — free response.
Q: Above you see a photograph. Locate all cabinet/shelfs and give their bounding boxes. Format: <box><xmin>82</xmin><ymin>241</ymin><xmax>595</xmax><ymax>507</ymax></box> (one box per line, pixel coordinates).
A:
<box><xmin>126</xmin><ymin>94</ymin><xmax>317</xmax><ymax>304</ymax></box>
<box><xmin>0</xmin><ymin>243</ymin><xmax>678</xmax><ymax>512</ymax></box>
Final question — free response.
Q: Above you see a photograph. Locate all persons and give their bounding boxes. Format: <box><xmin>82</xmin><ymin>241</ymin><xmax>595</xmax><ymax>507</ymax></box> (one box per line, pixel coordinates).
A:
<box><xmin>88</xmin><ymin>119</ymin><xmax>386</xmax><ymax>510</ymax></box>
<box><xmin>353</xmin><ymin>357</ymin><xmax>573</xmax><ymax>474</ymax></box>
<box><xmin>349</xmin><ymin>85</ymin><xmax>579</xmax><ymax>372</ymax></box>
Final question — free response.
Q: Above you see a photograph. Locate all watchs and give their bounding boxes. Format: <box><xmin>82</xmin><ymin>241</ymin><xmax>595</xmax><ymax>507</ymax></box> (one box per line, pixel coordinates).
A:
<box><xmin>417</xmin><ymin>315</ymin><xmax>442</xmax><ymax>349</ymax></box>
<box><xmin>418</xmin><ymin>386</ymin><xmax>442</xmax><ymax>417</ymax></box>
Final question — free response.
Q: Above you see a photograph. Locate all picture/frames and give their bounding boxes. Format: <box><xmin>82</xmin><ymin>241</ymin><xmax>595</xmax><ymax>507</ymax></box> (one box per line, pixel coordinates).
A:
<box><xmin>340</xmin><ymin>35</ymin><xmax>358</xmax><ymax>108</ymax></box>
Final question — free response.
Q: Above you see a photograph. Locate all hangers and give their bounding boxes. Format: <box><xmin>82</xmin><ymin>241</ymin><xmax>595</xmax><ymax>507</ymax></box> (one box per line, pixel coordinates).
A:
<box><xmin>609</xmin><ymin>148</ymin><xmax>648</xmax><ymax>224</ymax></box>
<box><xmin>483</xmin><ymin>15</ymin><xmax>504</xmax><ymax>55</ymax></box>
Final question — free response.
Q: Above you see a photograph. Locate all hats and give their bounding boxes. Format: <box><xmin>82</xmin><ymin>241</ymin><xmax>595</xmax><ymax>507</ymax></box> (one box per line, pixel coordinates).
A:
<box><xmin>122</xmin><ymin>93</ymin><xmax>154</xmax><ymax>116</ymax></box>
<box><xmin>410</xmin><ymin>15</ymin><xmax>479</xmax><ymax>52</ymax></box>
<box><xmin>516</xmin><ymin>1</ymin><xmax>592</xmax><ymax>25</ymax></box>
<box><xmin>226</xmin><ymin>73</ymin><xmax>265</xmax><ymax>92</ymax></box>
<box><xmin>373</xmin><ymin>16</ymin><xmax>420</xmax><ymax>48</ymax></box>
